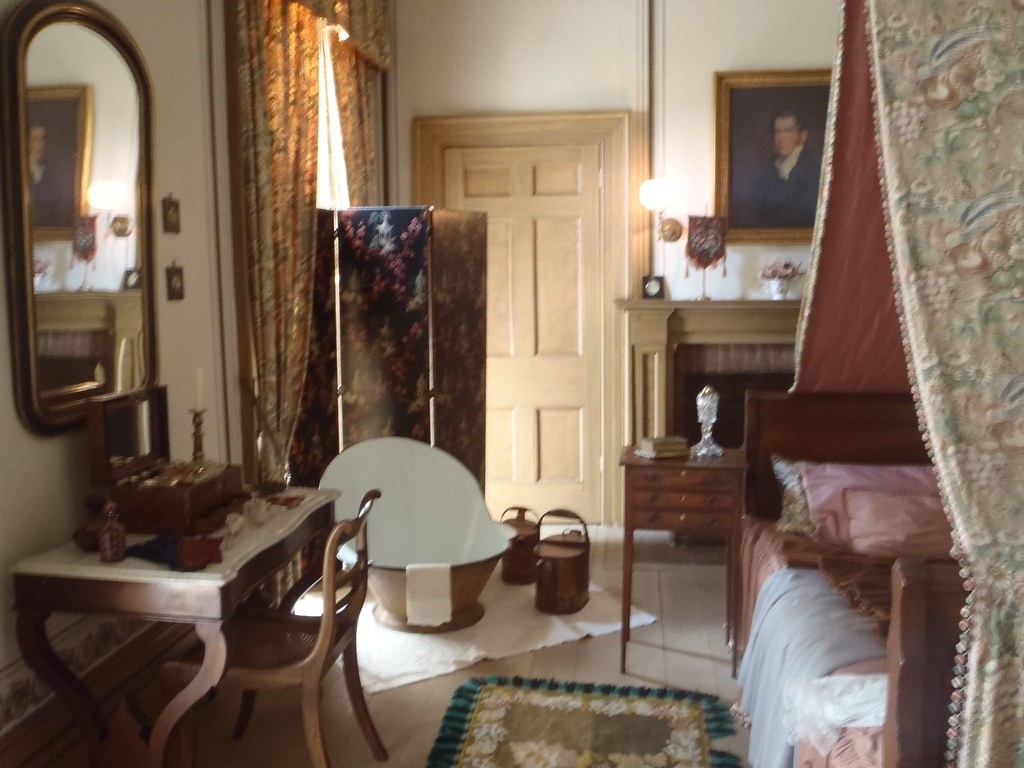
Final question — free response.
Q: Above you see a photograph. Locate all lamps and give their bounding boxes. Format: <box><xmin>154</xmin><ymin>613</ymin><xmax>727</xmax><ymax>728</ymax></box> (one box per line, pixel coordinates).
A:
<box><xmin>635</xmin><ymin>177</ymin><xmax>684</xmax><ymax>245</ymax></box>
<box><xmin>87</xmin><ymin>179</ymin><xmax>136</xmax><ymax>242</ymax></box>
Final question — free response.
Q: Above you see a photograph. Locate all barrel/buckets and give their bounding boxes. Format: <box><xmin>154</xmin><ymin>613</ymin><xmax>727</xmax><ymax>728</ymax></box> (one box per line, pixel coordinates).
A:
<box><xmin>503</xmin><ymin>505</ymin><xmax>540</xmax><ymax>586</ymax></box>
<box><xmin>531</xmin><ymin>507</ymin><xmax>595</xmax><ymax>618</ymax></box>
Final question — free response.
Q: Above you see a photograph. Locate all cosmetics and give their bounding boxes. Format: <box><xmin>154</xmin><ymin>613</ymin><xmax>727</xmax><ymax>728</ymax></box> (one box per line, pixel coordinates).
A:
<box><xmin>97</xmin><ymin>499</ymin><xmax>127</xmax><ymax>564</ymax></box>
<box><xmin>134</xmin><ymin>460</ymin><xmax>206</xmax><ymax>495</ymax></box>
<box><xmin>224</xmin><ymin>490</ymin><xmax>272</xmax><ymax>536</ymax></box>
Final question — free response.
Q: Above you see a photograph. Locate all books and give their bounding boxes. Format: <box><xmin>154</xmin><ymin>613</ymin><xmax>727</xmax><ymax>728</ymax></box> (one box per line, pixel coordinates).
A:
<box><xmin>634</xmin><ymin>435</ymin><xmax>690</xmax><ymax>461</ymax></box>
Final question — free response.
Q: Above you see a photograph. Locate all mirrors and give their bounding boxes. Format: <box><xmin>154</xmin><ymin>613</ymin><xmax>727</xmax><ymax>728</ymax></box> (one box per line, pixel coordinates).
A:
<box><xmin>1</xmin><ymin>0</ymin><xmax>163</xmax><ymax>437</ymax></box>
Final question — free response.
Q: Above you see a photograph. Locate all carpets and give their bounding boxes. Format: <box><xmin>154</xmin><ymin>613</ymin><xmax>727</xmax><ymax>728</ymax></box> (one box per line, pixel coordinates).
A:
<box><xmin>288</xmin><ymin>545</ymin><xmax>659</xmax><ymax>698</ymax></box>
<box><xmin>422</xmin><ymin>676</ymin><xmax>741</xmax><ymax>768</ymax></box>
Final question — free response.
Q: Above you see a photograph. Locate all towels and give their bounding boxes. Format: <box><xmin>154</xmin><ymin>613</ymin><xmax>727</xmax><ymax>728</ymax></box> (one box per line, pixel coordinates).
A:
<box><xmin>808</xmin><ymin>669</ymin><xmax>888</xmax><ymax>730</ymax></box>
<box><xmin>402</xmin><ymin>559</ymin><xmax>453</xmax><ymax>631</ymax></box>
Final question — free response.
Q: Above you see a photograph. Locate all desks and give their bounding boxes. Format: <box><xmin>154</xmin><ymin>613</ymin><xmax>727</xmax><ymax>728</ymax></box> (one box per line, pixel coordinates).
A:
<box><xmin>8</xmin><ymin>478</ymin><xmax>341</xmax><ymax>768</ymax></box>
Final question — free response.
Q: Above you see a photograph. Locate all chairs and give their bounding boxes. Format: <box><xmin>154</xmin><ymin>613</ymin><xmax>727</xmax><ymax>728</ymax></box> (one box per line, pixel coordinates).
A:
<box><xmin>160</xmin><ymin>488</ymin><xmax>392</xmax><ymax>768</ymax></box>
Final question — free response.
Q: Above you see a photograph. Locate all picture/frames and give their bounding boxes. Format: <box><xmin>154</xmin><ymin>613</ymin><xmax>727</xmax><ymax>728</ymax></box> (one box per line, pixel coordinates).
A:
<box><xmin>22</xmin><ymin>82</ymin><xmax>93</xmax><ymax>243</ymax></box>
<box><xmin>710</xmin><ymin>67</ymin><xmax>833</xmax><ymax>249</ymax></box>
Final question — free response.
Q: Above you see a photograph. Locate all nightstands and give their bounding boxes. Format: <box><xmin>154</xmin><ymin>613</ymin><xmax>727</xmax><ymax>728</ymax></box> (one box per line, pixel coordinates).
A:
<box><xmin>613</xmin><ymin>442</ymin><xmax>753</xmax><ymax>686</ymax></box>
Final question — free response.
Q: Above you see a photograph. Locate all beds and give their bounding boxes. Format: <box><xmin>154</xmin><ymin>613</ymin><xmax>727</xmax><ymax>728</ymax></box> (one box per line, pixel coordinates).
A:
<box><xmin>732</xmin><ymin>391</ymin><xmax>973</xmax><ymax>768</ymax></box>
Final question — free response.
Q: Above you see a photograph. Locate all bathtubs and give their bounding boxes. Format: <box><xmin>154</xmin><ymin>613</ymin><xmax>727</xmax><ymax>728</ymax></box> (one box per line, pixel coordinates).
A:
<box><xmin>313</xmin><ymin>433</ymin><xmax>514</xmax><ymax>634</ymax></box>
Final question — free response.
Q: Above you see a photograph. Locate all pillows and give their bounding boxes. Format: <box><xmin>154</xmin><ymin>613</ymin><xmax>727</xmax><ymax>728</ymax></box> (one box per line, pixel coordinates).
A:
<box><xmin>765</xmin><ymin>454</ymin><xmax>939</xmax><ymax>541</ymax></box>
<box><xmin>841</xmin><ymin>487</ymin><xmax>961</xmax><ymax>561</ymax></box>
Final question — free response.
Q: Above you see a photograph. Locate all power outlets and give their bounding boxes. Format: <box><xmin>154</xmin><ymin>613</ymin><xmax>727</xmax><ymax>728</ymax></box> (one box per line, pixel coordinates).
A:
<box><xmin>163</xmin><ymin>195</ymin><xmax>183</xmax><ymax>236</ymax></box>
<box><xmin>165</xmin><ymin>264</ymin><xmax>185</xmax><ymax>303</ymax></box>
